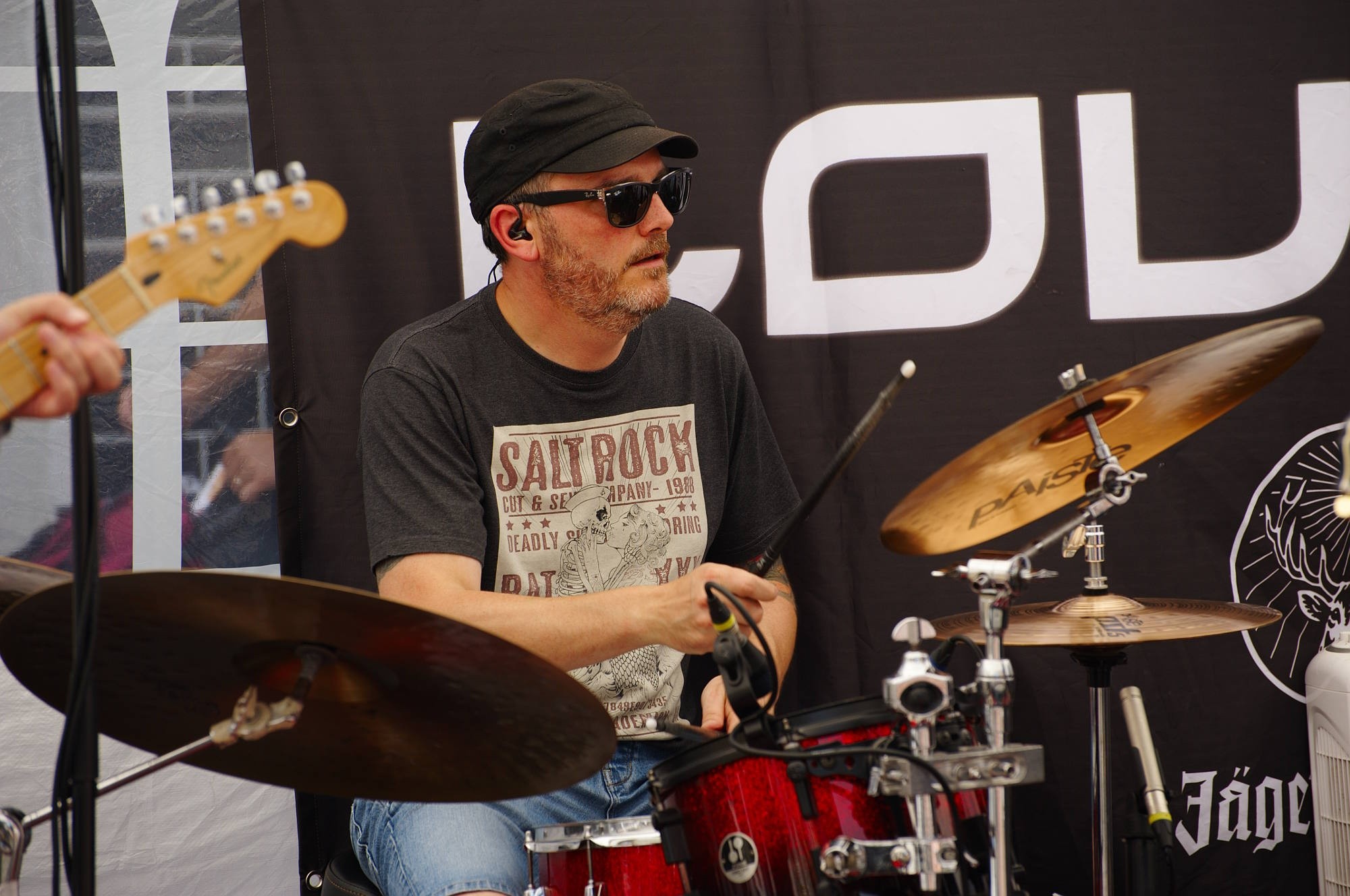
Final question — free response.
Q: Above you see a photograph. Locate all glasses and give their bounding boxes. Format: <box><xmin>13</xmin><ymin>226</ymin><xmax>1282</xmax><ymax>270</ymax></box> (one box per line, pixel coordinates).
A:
<box><xmin>502</xmin><ymin>167</ymin><xmax>693</xmax><ymax>226</ymax></box>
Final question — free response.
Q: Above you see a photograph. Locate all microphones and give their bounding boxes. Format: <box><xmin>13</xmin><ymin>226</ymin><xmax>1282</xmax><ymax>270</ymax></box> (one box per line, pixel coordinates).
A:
<box><xmin>1120</xmin><ymin>686</ymin><xmax>1173</xmax><ymax>849</ymax></box>
<box><xmin>706</xmin><ymin>593</ymin><xmax>773</xmax><ymax>698</ymax></box>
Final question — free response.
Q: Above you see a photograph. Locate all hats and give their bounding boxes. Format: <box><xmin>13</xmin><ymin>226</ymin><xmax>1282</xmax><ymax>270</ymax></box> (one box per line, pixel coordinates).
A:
<box><xmin>464</xmin><ymin>79</ymin><xmax>699</xmax><ymax>226</ymax></box>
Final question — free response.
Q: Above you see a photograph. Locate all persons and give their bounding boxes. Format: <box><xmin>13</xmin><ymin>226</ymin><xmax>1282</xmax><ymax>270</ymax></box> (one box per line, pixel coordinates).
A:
<box><xmin>350</xmin><ymin>79</ymin><xmax>804</xmax><ymax>896</ymax></box>
<box><xmin>118</xmin><ymin>264</ymin><xmax>281</xmax><ymax>569</ymax></box>
<box><xmin>0</xmin><ymin>290</ymin><xmax>124</xmax><ymax>419</ymax></box>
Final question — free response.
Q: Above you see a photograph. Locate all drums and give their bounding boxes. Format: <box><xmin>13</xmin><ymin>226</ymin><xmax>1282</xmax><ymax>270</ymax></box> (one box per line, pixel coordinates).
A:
<box><xmin>519</xmin><ymin>813</ymin><xmax>686</xmax><ymax>895</ymax></box>
<box><xmin>642</xmin><ymin>703</ymin><xmax>1020</xmax><ymax>895</ymax></box>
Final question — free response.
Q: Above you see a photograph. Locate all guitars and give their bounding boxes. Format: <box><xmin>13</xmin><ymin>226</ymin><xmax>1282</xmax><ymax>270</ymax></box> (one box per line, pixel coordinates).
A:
<box><xmin>3</xmin><ymin>157</ymin><xmax>349</xmax><ymax>420</ymax></box>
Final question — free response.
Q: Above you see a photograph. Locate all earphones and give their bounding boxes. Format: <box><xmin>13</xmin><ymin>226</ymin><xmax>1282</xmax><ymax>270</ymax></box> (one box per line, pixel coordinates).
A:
<box><xmin>509</xmin><ymin>217</ymin><xmax>533</xmax><ymax>242</ymax></box>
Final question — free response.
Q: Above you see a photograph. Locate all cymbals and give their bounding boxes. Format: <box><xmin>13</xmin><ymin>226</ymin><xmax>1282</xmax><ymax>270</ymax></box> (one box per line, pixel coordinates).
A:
<box><xmin>1</xmin><ymin>569</ymin><xmax>625</xmax><ymax>805</ymax></box>
<box><xmin>926</xmin><ymin>593</ymin><xmax>1288</xmax><ymax>651</ymax></box>
<box><xmin>878</xmin><ymin>310</ymin><xmax>1325</xmax><ymax>558</ymax></box>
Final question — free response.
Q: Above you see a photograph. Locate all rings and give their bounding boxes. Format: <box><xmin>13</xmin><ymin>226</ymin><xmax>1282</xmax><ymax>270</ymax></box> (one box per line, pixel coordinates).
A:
<box><xmin>231</xmin><ymin>477</ymin><xmax>244</xmax><ymax>487</ymax></box>
